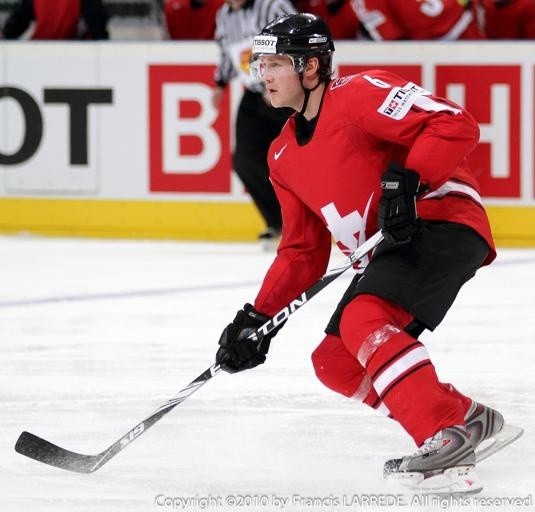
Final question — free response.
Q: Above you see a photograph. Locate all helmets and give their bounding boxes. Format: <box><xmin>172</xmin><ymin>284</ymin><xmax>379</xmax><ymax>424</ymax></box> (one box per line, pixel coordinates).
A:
<box><xmin>251</xmin><ymin>12</ymin><xmax>335</xmax><ymax>60</ymax></box>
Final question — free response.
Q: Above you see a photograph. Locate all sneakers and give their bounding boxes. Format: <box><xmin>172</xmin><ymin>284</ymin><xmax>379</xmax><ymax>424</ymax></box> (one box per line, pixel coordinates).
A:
<box><xmin>383</xmin><ymin>424</ymin><xmax>478</xmax><ymax>475</ymax></box>
<box><xmin>463</xmin><ymin>399</ymin><xmax>506</xmax><ymax>449</ymax></box>
<box><xmin>258</xmin><ymin>227</ymin><xmax>282</xmax><ymax>239</ymax></box>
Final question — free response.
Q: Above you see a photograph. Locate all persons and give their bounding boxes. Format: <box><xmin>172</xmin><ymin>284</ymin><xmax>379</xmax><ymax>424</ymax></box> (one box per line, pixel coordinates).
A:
<box><xmin>214</xmin><ymin>12</ymin><xmax>505</xmax><ymax>480</ymax></box>
<box><xmin>211</xmin><ymin>0</ymin><xmax>302</xmax><ymax>238</ymax></box>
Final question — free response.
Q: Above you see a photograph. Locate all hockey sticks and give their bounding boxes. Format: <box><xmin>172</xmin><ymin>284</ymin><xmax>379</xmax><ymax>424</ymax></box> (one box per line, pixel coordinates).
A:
<box><xmin>16</xmin><ymin>225</ymin><xmax>381</xmax><ymax>475</ymax></box>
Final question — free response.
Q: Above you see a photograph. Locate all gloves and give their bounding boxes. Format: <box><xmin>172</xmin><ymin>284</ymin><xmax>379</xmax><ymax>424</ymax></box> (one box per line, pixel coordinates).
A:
<box><xmin>215</xmin><ymin>299</ymin><xmax>290</xmax><ymax>374</ymax></box>
<box><xmin>377</xmin><ymin>158</ymin><xmax>432</xmax><ymax>247</ymax></box>
